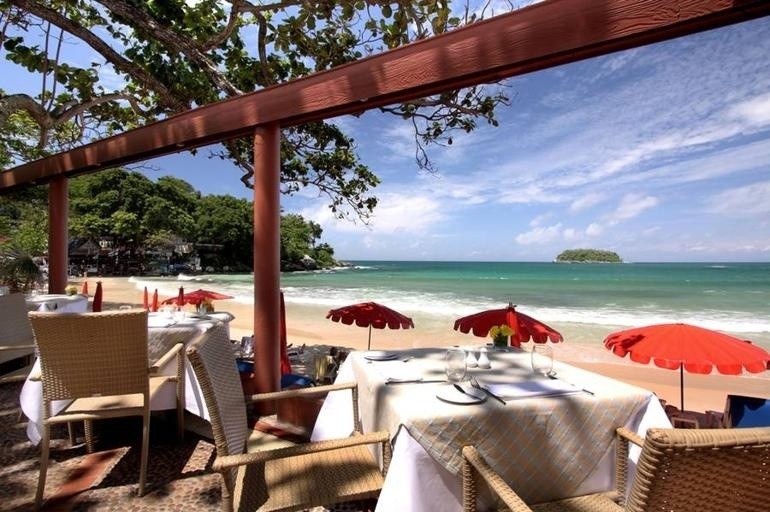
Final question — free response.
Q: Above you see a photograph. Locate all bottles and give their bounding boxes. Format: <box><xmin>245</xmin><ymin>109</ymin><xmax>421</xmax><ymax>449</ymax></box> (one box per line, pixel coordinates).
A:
<box><xmin>466</xmin><ymin>348</ymin><xmax>479</xmax><ymax>370</ymax></box>
<box><xmin>478</xmin><ymin>348</ymin><xmax>491</xmax><ymax>368</ymax></box>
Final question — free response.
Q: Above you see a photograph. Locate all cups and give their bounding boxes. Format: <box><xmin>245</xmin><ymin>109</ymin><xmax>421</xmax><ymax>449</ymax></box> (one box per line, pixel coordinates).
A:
<box><xmin>200</xmin><ymin>304</ymin><xmax>207</xmax><ymax>315</ymax></box>
<box><xmin>239</xmin><ymin>336</ymin><xmax>253</xmax><ymax>358</ymax></box>
<box><xmin>531</xmin><ymin>344</ymin><xmax>553</xmax><ymax>376</ymax></box>
<box><xmin>444</xmin><ymin>348</ymin><xmax>467</xmax><ymax>384</ymax></box>
<box><xmin>31</xmin><ymin>289</ymin><xmax>36</xmax><ymax>297</ymax></box>
<box><xmin>160</xmin><ymin>304</ymin><xmax>178</xmax><ymax>320</ymax></box>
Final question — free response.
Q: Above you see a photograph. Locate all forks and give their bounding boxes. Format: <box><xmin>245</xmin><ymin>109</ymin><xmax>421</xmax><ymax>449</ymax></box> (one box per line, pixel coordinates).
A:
<box><xmin>467</xmin><ymin>376</ymin><xmax>506</xmax><ymax>406</ymax></box>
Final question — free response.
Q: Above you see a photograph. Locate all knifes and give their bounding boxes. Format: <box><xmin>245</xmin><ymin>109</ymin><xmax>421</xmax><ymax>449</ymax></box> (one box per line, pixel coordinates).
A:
<box><xmin>386</xmin><ymin>378</ymin><xmax>447</xmax><ymax>385</ymax></box>
<box><xmin>452</xmin><ymin>384</ymin><xmax>482</xmax><ymax>402</ymax></box>
<box><xmin>549</xmin><ymin>374</ymin><xmax>595</xmax><ymax>397</ymax></box>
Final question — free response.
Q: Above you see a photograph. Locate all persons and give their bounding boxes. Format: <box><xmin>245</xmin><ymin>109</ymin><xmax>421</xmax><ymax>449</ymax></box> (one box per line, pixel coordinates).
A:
<box><xmin>79</xmin><ymin>259</ymin><xmax>197</xmax><ymax>277</ymax></box>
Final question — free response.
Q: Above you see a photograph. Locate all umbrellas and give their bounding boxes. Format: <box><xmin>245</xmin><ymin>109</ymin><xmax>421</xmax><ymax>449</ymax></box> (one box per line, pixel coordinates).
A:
<box><xmin>326</xmin><ymin>300</ymin><xmax>414</xmax><ymax>349</ymax></box>
<box><xmin>82</xmin><ymin>280</ymin><xmax>89</xmax><ymax>294</ymax></box>
<box><xmin>143</xmin><ymin>286</ymin><xmax>150</xmax><ymax>311</ymax></box>
<box><xmin>454</xmin><ymin>303</ymin><xmax>563</xmax><ymax>349</ymax></box>
<box><xmin>177</xmin><ymin>285</ymin><xmax>185</xmax><ymax>307</ymax></box>
<box><xmin>280</xmin><ymin>292</ymin><xmax>291</xmax><ymax>373</ymax></box>
<box><xmin>162</xmin><ymin>288</ymin><xmax>234</xmax><ymax>311</ymax></box>
<box><xmin>603</xmin><ymin>322</ymin><xmax>769</xmax><ymax>412</ymax></box>
<box><xmin>93</xmin><ymin>280</ymin><xmax>104</xmax><ymax>312</ymax></box>
<box><xmin>152</xmin><ymin>289</ymin><xmax>159</xmax><ymax>312</ymax></box>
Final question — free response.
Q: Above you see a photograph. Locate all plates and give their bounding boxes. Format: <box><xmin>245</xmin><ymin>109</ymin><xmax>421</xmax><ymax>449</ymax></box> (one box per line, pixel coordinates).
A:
<box><xmin>435</xmin><ymin>383</ymin><xmax>487</xmax><ymax>405</ymax></box>
<box><xmin>147</xmin><ymin>311</ymin><xmax>177</xmax><ymax>329</ymax></box>
<box><xmin>361</xmin><ymin>350</ymin><xmax>398</xmax><ymax>361</ymax></box>
<box><xmin>187</xmin><ymin>315</ymin><xmax>213</xmax><ymax>321</ymax></box>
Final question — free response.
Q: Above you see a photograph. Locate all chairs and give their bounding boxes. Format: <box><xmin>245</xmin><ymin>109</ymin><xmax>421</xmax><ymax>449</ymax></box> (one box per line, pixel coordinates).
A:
<box><xmin>0</xmin><ymin>293</ymin><xmax>37</xmax><ymax>418</ymax></box>
<box><xmin>28</xmin><ymin>311</ymin><xmax>184</xmax><ymax>512</ymax></box>
<box><xmin>187</xmin><ymin>322</ymin><xmax>389</xmax><ymax>512</ymax></box>
<box><xmin>659</xmin><ymin>398</ymin><xmax>723</xmax><ymax>428</ymax></box>
<box><xmin>462</xmin><ymin>427</ymin><xmax>770</xmax><ymax>512</ymax></box>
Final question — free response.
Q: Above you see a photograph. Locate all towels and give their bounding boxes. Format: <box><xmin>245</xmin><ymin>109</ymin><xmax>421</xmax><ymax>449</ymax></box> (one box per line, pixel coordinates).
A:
<box><xmin>485</xmin><ymin>379</ymin><xmax>579</xmax><ymax>399</ymax></box>
<box><xmin>147</xmin><ymin>317</ymin><xmax>176</xmax><ymax>327</ymax></box>
<box><xmin>373</xmin><ymin>360</ymin><xmax>423</xmax><ymax>382</ymax></box>
